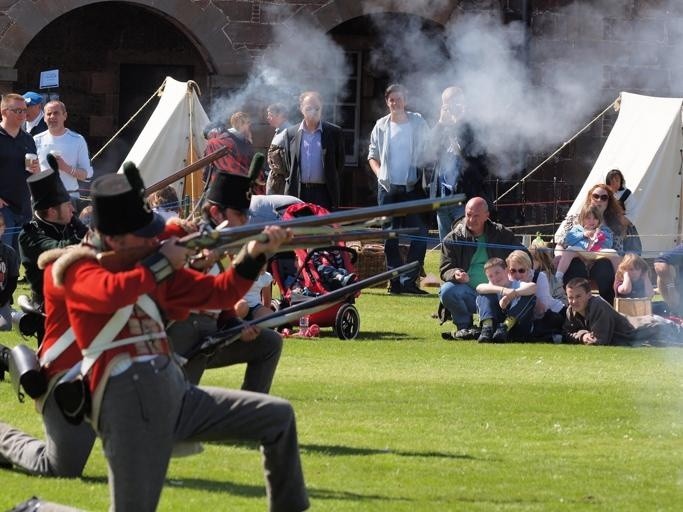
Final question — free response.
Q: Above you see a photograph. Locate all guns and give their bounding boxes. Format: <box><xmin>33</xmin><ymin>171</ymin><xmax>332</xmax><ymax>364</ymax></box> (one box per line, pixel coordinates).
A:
<box><xmin>97</xmin><ymin>192</ymin><xmax>467</xmax><ymax>274</ymax></box>
<box><xmin>144</xmin><ymin>145</ymin><xmax>228</xmax><ymax>199</ymax></box>
<box><xmin>183</xmin><ymin>260</ymin><xmax>419</xmax><ymax>370</ymax></box>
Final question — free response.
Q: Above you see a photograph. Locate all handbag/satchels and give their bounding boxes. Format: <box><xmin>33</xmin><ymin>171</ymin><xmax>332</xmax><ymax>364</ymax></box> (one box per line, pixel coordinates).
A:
<box><xmin>614</xmin><ymin>296</ymin><xmax>653</xmax><ymax>317</ymax></box>
<box><xmin>13</xmin><ymin>310</ymin><xmax>38</xmax><ymax>342</ymax></box>
<box><xmin>8</xmin><ymin>344</ymin><xmax>48</xmax><ymax>403</ymax></box>
<box><xmin>52</xmin><ymin>359</ymin><xmax>92</xmax><ymax>426</ymax></box>
<box><xmin>652</xmin><ymin>300</ymin><xmax>671</xmax><ymax>320</ymax></box>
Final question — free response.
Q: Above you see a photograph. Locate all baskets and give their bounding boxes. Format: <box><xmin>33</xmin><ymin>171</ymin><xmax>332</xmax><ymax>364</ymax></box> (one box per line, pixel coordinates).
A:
<box><xmin>347</xmin><ymin>245</ymin><xmax>387</xmax><ymax>288</ymax></box>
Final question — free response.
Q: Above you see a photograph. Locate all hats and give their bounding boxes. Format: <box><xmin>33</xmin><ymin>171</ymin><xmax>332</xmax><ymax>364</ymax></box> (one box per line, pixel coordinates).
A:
<box><xmin>205</xmin><ymin>152</ymin><xmax>265</xmax><ymax>209</ymax></box>
<box><xmin>25</xmin><ymin>153</ymin><xmax>70</xmax><ymax>211</ymax></box>
<box><xmin>89</xmin><ymin>161</ymin><xmax>165</xmax><ymax>238</ymax></box>
<box><xmin>21</xmin><ymin>91</ymin><xmax>44</xmax><ymax>106</ymax></box>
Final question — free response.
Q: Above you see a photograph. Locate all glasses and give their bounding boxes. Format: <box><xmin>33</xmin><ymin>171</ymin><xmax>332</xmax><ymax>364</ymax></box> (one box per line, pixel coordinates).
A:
<box><xmin>508</xmin><ymin>268</ymin><xmax>526</xmax><ymax>274</ymax></box>
<box><xmin>305</xmin><ymin>105</ymin><xmax>320</xmax><ymax>111</ymax></box>
<box><xmin>591</xmin><ymin>193</ymin><xmax>609</xmax><ymax>201</ymax></box>
<box><xmin>7</xmin><ymin>108</ymin><xmax>28</xmax><ymax>114</ymax></box>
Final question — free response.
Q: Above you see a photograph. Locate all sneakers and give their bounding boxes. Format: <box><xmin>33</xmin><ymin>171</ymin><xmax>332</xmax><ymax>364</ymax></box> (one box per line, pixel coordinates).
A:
<box><xmin>492</xmin><ymin>323</ymin><xmax>513</xmax><ymax>341</ymax></box>
<box><xmin>340</xmin><ymin>272</ymin><xmax>356</xmax><ymax>288</ymax></box>
<box><xmin>477</xmin><ymin>326</ymin><xmax>494</xmax><ymax>343</ymax></box>
<box><xmin>0</xmin><ymin>300</ymin><xmax>13</xmax><ymax>331</ymax></box>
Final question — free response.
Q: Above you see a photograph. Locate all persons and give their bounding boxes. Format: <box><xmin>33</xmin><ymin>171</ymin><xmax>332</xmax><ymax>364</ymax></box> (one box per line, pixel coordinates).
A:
<box><xmin>0</xmin><ymin>202</ymin><xmax>218</xmax><ymax>478</ymax></box>
<box><xmin>1</xmin><ymin>91</ymin><xmax>91</xmax><ymax>333</ymax></box>
<box><xmin>441</xmin><ymin>170</ymin><xmax>682</xmax><ymax>347</ymax></box>
<box><xmin>368</xmin><ymin>83</ymin><xmax>495</xmax><ymax>295</ymax></box>
<box><xmin>151</xmin><ymin>92</ymin><xmax>357</xmax><ymax>338</ymax></box>
<box><xmin>160</xmin><ymin>172</ymin><xmax>285</xmax><ymax>395</ymax></box>
<box><xmin>51</xmin><ymin>174</ymin><xmax>311</xmax><ymax>512</ymax></box>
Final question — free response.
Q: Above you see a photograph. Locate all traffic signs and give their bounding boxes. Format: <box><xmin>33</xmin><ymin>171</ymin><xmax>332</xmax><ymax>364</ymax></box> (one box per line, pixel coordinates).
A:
<box><xmin>36</xmin><ymin>68</ymin><xmax>59</xmax><ymax>90</ymax></box>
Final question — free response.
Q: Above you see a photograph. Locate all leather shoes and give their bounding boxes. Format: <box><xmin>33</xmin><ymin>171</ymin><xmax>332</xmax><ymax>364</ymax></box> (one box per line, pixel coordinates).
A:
<box><xmin>401</xmin><ymin>285</ymin><xmax>430</xmax><ymax>297</ymax></box>
<box><xmin>390</xmin><ymin>284</ymin><xmax>401</xmax><ymax>294</ymax></box>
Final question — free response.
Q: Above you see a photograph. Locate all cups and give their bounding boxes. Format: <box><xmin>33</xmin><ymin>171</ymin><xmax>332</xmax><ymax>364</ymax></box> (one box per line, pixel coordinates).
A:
<box><xmin>50</xmin><ymin>151</ymin><xmax>60</xmax><ymax>159</ymax></box>
<box><xmin>24</xmin><ymin>153</ymin><xmax>37</xmax><ymax>172</ymax></box>
<box><xmin>522</xmin><ymin>235</ymin><xmax>530</xmax><ymax>247</ymax></box>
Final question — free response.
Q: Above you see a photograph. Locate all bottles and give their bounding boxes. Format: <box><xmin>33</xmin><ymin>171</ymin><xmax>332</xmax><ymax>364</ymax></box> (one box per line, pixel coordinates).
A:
<box><xmin>298</xmin><ymin>316</ymin><xmax>308</xmax><ymax>332</ymax></box>
<box><xmin>530</xmin><ymin>232</ymin><xmax>544</xmax><ymax>247</ymax></box>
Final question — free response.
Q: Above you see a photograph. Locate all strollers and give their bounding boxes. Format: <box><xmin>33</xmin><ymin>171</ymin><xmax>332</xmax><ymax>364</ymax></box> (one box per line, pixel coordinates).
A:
<box><xmin>263</xmin><ymin>202</ymin><xmax>361</xmax><ymax>342</ymax></box>
